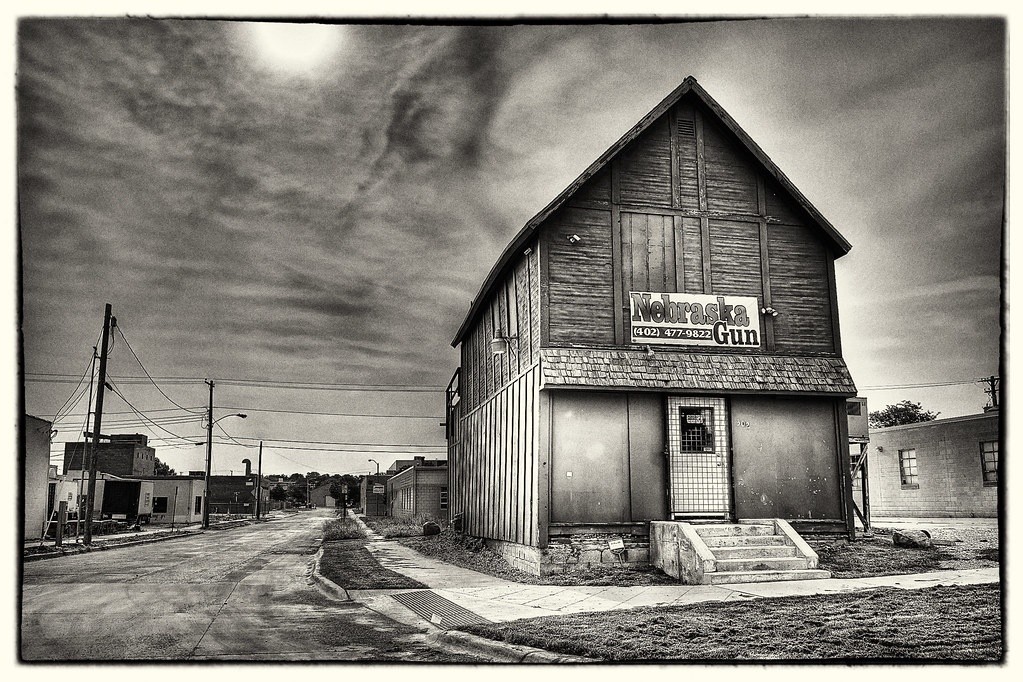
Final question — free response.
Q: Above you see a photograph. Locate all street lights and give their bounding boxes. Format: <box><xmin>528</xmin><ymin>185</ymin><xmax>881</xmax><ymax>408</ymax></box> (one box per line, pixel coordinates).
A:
<box><xmin>368</xmin><ymin>459</ymin><xmax>379</xmax><ymax>516</ymax></box>
<box><xmin>202</xmin><ymin>413</ymin><xmax>247</xmax><ymax>526</ymax></box>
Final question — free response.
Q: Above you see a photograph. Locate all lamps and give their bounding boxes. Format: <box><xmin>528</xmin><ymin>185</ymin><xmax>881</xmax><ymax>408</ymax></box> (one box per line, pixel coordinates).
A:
<box><xmin>567</xmin><ymin>235</ymin><xmax>579</xmax><ymax>244</ymax></box>
<box><xmin>524</xmin><ymin>248</ymin><xmax>532</xmax><ymax>255</ymax></box>
<box><xmin>760</xmin><ymin>307</ymin><xmax>778</xmax><ymax>317</ymax></box>
<box><xmin>492</xmin><ymin>328</ymin><xmax>517</xmax><ymax>354</ymax></box>
<box><xmin>646</xmin><ymin>345</ymin><xmax>655</xmax><ymax>356</ymax></box>
